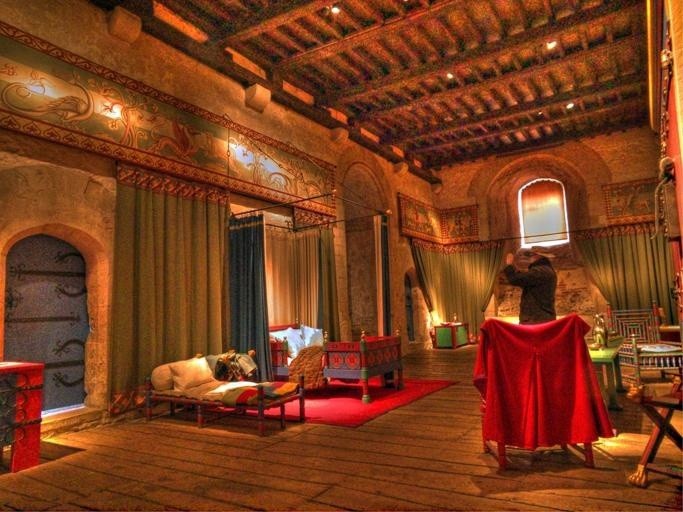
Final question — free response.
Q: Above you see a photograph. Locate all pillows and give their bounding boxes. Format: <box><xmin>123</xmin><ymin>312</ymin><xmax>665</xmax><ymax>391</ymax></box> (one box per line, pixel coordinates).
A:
<box><xmin>170</xmin><ymin>359</ymin><xmax>214</xmax><ymax>390</ymax></box>
<box><xmin>301</xmin><ymin>325</ymin><xmax>326</xmax><ymax>351</ymax></box>
<box><xmin>273</xmin><ymin>326</ymin><xmax>306</xmax><ymax>357</ymax></box>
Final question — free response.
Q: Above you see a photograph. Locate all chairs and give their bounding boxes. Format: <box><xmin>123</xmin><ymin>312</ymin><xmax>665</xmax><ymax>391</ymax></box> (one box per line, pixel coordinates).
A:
<box><xmin>476</xmin><ymin>319</ymin><xmax>593</xmax><ymax>466</ymax></box>
<box><xmin>607</xmin><ymin>305</ymin><xmax>681</xmax><ymax>381</ymax></box>
<box><xmin>626</xmin><ymin>367</ymin><xmax>682</xmax><ymax>484</ymax></box>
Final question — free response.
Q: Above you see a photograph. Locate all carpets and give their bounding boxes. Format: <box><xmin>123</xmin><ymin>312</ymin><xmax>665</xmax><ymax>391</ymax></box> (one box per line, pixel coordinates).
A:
<box><xmin>209</xmin><ymin>370</ymin><xmax>459</xmax><ymax>430</ymax></box>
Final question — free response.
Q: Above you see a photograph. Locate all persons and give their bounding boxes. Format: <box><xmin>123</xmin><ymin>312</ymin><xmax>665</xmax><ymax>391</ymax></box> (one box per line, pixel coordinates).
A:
<box><xmin>503</xmin><ymin>246</ymin><xmax>557</xmax><ymax>326</ymax></box>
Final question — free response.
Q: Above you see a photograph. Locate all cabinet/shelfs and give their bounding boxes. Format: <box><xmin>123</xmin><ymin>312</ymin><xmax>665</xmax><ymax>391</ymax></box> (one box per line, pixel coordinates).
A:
<box><xmin>433</xmin><ymin>322</ymin><xmax>467</xmax><ymax>351</ymax></box>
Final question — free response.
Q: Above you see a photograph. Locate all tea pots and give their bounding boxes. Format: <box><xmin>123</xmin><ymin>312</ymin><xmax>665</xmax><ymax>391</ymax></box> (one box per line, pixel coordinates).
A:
<box><xmin>592</xmin><ymin>314</ymin><xmax>609</xmax><ymax>350</ymax></box>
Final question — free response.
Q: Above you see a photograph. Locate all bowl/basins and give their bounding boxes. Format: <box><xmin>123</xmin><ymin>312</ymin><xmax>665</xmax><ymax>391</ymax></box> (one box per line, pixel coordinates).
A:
<box><xmin>587</xmin><ymin>343</ymin><xmax>601</xmax><ymax>351</ymax></box>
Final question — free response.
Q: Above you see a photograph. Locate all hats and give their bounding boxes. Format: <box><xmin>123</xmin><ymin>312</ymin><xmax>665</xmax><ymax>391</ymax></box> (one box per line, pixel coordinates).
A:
<box><xmin>517</xmin><ymin>245</ymin><xmax>556</xmax><ymax>259</ymax></box>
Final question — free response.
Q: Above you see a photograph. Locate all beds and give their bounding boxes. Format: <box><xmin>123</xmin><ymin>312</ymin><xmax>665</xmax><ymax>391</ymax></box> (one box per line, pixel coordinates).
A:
<box><xmin>139</xmin><ymin>358</ymin><xmax>305</xmax><ymax>432</ymax></box>
<box><xmin>261</xmin><ymin>323</ymin><xmax>405</xmax><ymax>406</ymax></box>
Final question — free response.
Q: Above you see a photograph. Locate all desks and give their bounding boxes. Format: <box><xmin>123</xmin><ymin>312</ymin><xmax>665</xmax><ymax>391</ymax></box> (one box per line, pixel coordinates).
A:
<box><xmin>581</xmin><ymin>341</ymin><xmax>628</xmax><ymax>412</ymax></box>
